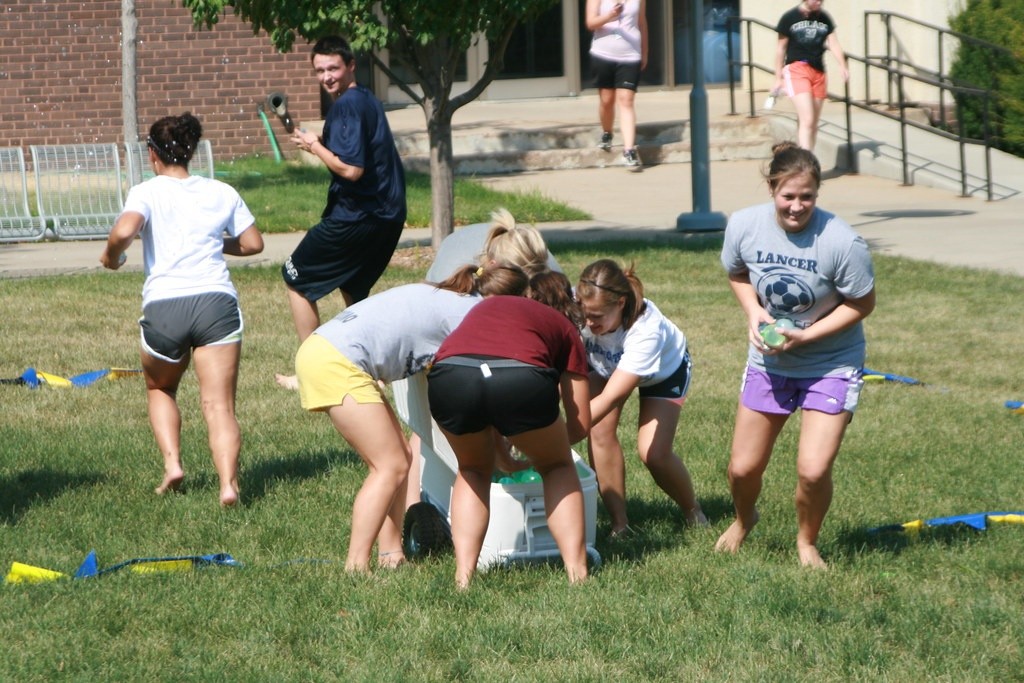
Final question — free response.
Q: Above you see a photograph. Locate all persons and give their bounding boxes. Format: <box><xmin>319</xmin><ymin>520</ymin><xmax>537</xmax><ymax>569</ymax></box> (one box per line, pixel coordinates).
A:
<box><xmin>100</xmin><ymin>112</ymin><xmax>268</xmax><ymax>502</ymax></box>
<box><xmin>426</xmin><ymin>269</ymin><xmax>600</xmax><ymax>598</ymax></box>
<box><xmin>279</xmin><ymin>38</ymin><xmax>408</xmax><ymax>391</ymax></box>
<box><xmin>426</xmin><ymin>206</ymin><xmax>556</xmax><ymax>279</ymax></box>
<box><xmin>294</xmin><ymin>249</ymin><xmax>532</xmax><ymax>576</ymax></box>
<box><xmin>559</xmin><ymin>258</ymin><xmax>712</xmax><ymax>540</ymax></box>
<box><xmin>581</xmin><ymin>1</ymin><xmax>649</xmax><ymax>172</ymax></box>
<box><xmin>719</xmin><ymin>139</ymin><xmax>880</xmax><ymax>572</ymax></box>
<box><xmin>769</xmin><ymin>0</ymin><xmax>850</xmax><ymax>150</ymax></box>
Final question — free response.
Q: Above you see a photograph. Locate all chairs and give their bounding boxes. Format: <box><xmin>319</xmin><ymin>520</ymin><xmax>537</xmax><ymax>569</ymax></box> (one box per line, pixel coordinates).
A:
<box><xmin>1</xmin><ymin>146</ymin><xmax>46</xmax><ymax>242</ymax></box>
<box><xmin>30</xmin><ymin>142</ymin><xmax>126</xmax><ymax>240</ymax></box>
<box><xmin>125</xmin><ymin>139</ymin><xmax>215</xmax><ymax>239</ymax></box>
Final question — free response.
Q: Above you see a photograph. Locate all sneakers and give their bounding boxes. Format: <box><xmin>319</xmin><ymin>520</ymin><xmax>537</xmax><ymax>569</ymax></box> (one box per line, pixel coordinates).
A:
<box><xmin>621</xmin><ymin>145</ymin><xmax>643</xmax><ymax>172</ymax></box>
<box><xmin>600</xmin><ymin>132</ymin><xmax>612</xmax><ymax>150</ymax></box>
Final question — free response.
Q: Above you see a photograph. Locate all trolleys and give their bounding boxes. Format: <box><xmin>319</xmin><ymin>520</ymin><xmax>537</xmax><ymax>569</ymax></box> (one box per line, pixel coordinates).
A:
<box><xmin>402</xmin><ymin>429</ymin><xmax>604</xmax><ymax>575</ymax></box>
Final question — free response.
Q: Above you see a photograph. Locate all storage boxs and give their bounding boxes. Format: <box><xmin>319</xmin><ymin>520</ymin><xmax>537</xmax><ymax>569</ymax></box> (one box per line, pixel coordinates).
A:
<box><xmin>401</xmin><ymin>435</ymin><xmax>601</xmax><ymax>577</ymax></box>
